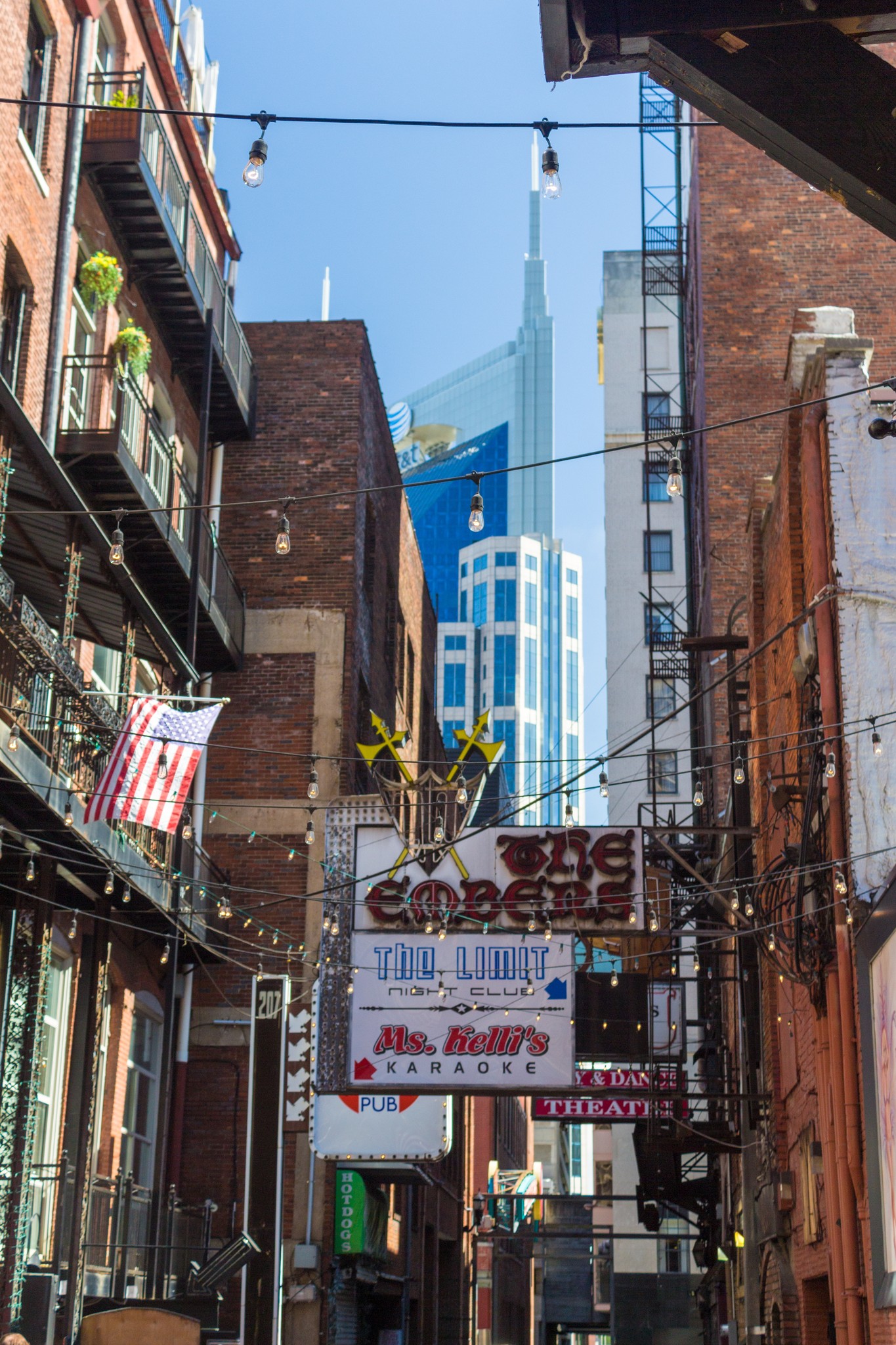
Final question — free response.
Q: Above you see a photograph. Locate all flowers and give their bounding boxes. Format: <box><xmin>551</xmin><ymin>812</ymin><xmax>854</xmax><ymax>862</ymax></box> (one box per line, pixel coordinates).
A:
<box><xmin>97</xmin><ymin>88</ymin><xmax>141</xmax><ymax>111</ymax></box>
<box><xmin>112</xmin><ymin>316</ymin><xmax>154</xmax><ymax>380</ymax></box>
<box><xmin>80</xmin><ymin>247</ymin><xmax>126</xmax><ymax>312</ymax></box>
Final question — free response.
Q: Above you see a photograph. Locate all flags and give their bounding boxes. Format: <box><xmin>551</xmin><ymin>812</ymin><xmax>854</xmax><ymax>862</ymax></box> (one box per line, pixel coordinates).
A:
<box><xmin>84</xmin><ymin>694</ymin><xmax>226</xmax><ymax>835</ymax></box>
